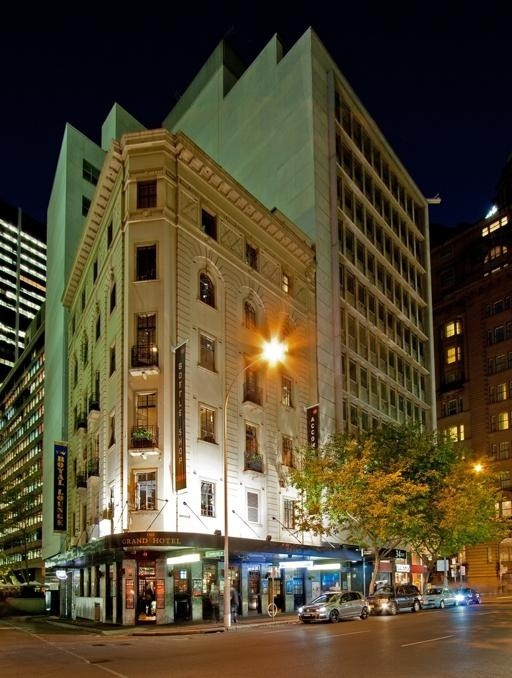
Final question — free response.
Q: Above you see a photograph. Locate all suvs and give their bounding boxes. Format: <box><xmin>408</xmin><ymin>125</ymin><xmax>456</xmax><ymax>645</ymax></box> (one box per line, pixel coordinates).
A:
<box><xmin>367</xmin><ymin>585</ymin><xmax>424</xmax><ymax>615</ymax></box>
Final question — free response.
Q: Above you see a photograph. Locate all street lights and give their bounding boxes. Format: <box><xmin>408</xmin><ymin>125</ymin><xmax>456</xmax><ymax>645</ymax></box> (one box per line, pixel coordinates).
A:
<box><xmin>223</xmin><ymin>344</ymin><xmax>283</xmax><ymax>629</ymax></box>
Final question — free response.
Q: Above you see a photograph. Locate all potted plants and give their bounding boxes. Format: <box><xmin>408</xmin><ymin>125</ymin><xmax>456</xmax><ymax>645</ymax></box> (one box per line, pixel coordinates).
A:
<box><xmin>132</xmin><ymin>431</ymin><xmax>152</xmax><ymax>447</ymax></box>
<box><xmin>245</xmin><ymin>451</ymin><xmax>263</xmax><ymax>472</ymax></box>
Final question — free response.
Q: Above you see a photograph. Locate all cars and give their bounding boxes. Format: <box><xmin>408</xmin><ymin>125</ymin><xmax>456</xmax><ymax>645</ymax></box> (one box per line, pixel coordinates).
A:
<box><xmin>298</xmin><ymin>591</ymin><xmax>369</xmax><ymax>622</ymax></box>
<box><xmin>454</xmin><ymin>588</ymin><xmax>481</xmax><ymax>606</ymax></box>
<box><xmin>421</xmin><ymin>587</ymin><xmax>459</xmax><ymax>609</ymax></box>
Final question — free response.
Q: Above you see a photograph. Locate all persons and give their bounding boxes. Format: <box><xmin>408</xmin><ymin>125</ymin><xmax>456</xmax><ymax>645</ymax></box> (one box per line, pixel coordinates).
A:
<box><xmin>207</xmin><ymin>585</ymin><xmax>221</xmax><ymax>624</ymax></box>
<box><xmin>229</xmin><ymin>586</ymin><xmax>240</xmax><ymax>623</ymax></box>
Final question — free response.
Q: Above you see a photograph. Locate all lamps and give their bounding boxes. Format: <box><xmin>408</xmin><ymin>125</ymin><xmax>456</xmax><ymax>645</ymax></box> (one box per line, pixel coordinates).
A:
<box><xmin>200</xmin><ymin>280</ymin><xmax>209</xmax><ymax>299</ymax></box>
